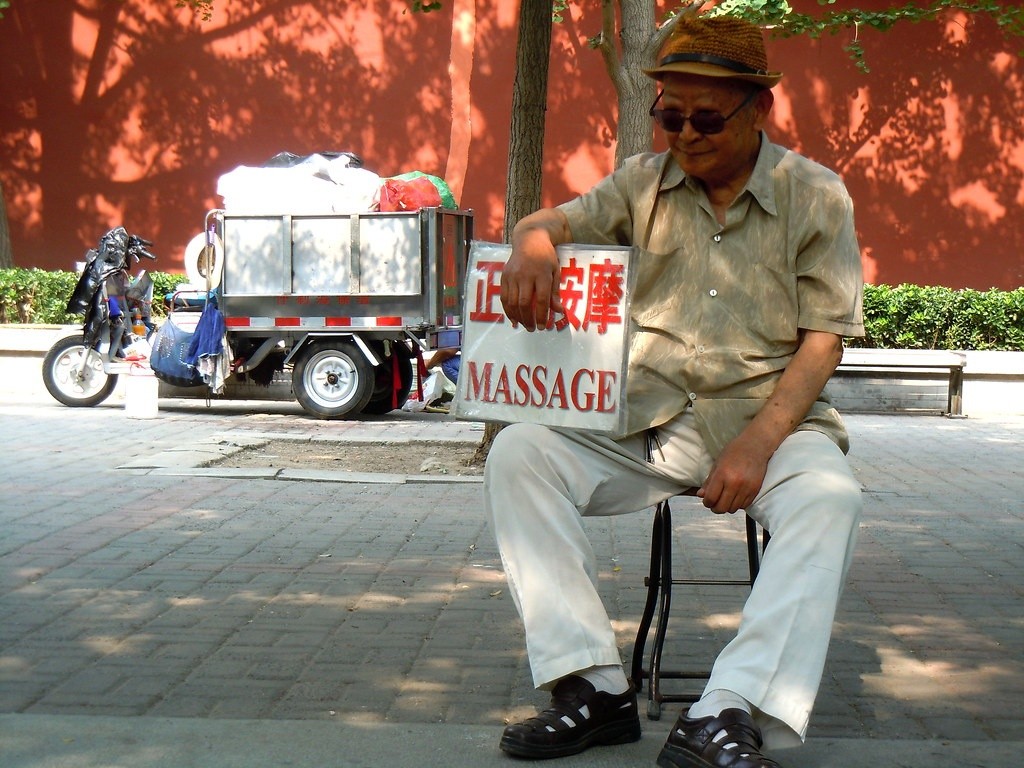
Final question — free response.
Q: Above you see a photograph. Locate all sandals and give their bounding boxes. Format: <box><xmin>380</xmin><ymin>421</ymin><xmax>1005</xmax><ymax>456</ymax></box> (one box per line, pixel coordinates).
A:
<box><xmin>656</xmin><ymin>707</ymin><xmax>782</xmax><ymax>768</ymax></box>
<box><xmin>498</xmin><ymin>674</ymin><xmax>641</xmax><ymax>761</ymax></box>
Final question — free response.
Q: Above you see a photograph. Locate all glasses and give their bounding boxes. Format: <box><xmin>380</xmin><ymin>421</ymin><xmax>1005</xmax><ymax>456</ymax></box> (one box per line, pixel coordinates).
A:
<box><xmin>649</xmin><ymin>86</ymin><xmax>756</xmax><ymax>136</ymax></box>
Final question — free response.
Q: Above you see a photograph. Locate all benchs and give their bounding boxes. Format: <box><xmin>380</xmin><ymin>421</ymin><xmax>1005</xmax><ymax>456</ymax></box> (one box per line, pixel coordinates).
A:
<box><xmin>824</xmin><ymin>345</ymin><xmax>967</xmax><ymax>419</ymax></box>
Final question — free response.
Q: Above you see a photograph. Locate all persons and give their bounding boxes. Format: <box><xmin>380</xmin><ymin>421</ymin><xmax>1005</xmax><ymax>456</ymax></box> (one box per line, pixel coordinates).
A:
<box><xmin>483</xmin><ymin>12</ymin><xmax>864</xmax><ymax>768</ymax></box>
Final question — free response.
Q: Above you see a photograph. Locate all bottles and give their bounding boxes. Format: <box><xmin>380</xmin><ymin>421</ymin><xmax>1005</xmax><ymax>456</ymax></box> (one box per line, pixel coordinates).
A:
<box><xmin>133</xmin><ymin>306</ymin><xmax>140</xmax><ymax>312</ymax></box>
<box><xmin>132</xmin><ymin>315</ymin><xmax>146</xmax><ymax>340</ymax></box>
<box><xmin>125</xmin><ymin>369</ymin><xmax>159</xmax><ymax>419</ymax></box>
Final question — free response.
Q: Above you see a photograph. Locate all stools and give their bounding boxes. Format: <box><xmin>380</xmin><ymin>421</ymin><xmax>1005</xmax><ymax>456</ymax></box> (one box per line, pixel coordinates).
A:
<box><xmin>630</xmin><ymin>490</ymin><xmax>770</xmax><ymax>721</ymax></box>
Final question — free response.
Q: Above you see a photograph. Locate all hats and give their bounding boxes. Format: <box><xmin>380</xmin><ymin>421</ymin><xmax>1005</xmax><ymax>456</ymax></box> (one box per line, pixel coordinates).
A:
<box><xmin>640</xmin><ymin>17</ymin><xmax>783</xmax><ymax>90</ymax></box>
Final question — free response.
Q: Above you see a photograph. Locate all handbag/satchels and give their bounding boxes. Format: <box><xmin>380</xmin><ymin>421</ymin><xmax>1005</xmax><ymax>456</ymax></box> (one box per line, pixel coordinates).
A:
<box><xmin>150</xmin><ymin>320</ymin><xmax>204</xmax><ymax>386</ymax></box>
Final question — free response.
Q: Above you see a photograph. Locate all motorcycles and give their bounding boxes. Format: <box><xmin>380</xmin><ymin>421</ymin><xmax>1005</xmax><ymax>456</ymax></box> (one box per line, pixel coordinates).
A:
<box><xmin>42</xmin><ymin>207</ymin><xmax>473</xmax><ymax>424</ymax></box>
